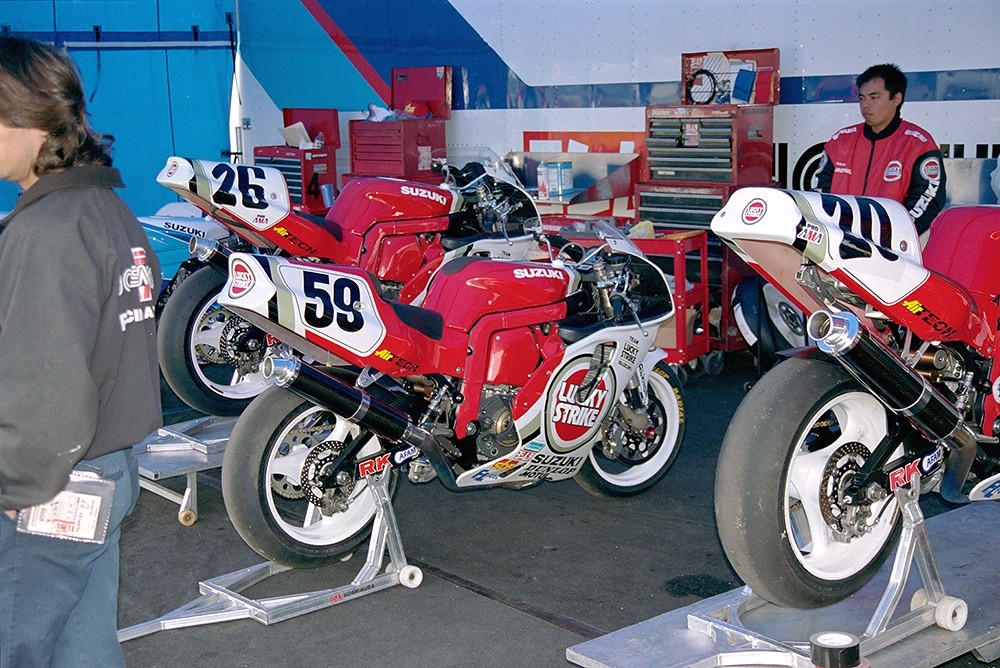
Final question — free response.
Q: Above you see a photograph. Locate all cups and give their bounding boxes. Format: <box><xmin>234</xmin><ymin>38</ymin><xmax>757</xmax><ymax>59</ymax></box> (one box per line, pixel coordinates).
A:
<box><xmin>320</xmin><ymin>184</ymin><xmax>336</xmax><ymax>208</ymax></box>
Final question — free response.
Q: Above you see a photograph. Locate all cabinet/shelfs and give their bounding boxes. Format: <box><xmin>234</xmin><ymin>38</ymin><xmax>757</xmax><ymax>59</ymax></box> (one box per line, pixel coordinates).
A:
<box><xmin>569</xmin><ymin>101</ymin><xmax>773</xmax><ymax>365</ymax></box>
<box><xmin>253</xmin><ymin>120</ymin><xmax>447</xmax><ymax>217</ymax></box>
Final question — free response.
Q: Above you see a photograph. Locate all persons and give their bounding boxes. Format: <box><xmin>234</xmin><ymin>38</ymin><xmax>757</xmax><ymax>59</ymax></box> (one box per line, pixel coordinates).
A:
<box><xmin>0</xmin><ymin>34</ymin><xmax>165</xmax><ymax>667</ymax></box>
<box><xmin>809</xmin><ymin>63</ymin><xmax>948</xmax><ymax>237</ymax></box>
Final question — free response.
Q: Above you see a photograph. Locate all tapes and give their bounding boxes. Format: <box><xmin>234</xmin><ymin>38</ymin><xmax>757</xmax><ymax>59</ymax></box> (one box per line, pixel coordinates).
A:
<box><xmin>809</xmin><ymin>632</ymin><xmax>860</xmax><ymax>668</ymax></box>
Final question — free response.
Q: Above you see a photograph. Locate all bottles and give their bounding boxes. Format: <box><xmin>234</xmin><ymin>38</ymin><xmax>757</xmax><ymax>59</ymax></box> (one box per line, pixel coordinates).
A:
<box><xmin>536</xmin><ymin>159</ymin><xmax>550</xmax><ymax>200</ymax></box>
<box><xmin>312</xmin><ymin>130</ymin><xmax>324</xmax><ymax>149</ymax></box>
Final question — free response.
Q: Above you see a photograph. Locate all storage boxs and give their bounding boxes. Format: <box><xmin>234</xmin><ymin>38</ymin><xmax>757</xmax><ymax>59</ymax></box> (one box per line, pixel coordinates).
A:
<box><xmin>653</xmin><ymin>308</ymin><xmax>699</xmax><ymax>349</ymax></box>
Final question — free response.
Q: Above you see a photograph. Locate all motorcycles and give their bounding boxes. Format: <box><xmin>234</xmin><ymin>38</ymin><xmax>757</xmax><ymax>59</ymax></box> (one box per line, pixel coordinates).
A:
<box><xmin>708</xmin><ymin>181</ymin><xmax>1000</xmax><ymax>613</ymax></box>
<box><xmin>152</xmin><ymin>163</ymin><xmax>690</xmax><ymax>569</ymax></box>
